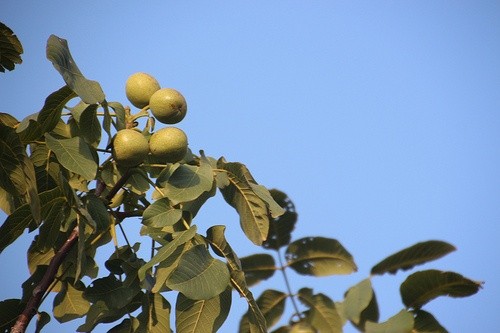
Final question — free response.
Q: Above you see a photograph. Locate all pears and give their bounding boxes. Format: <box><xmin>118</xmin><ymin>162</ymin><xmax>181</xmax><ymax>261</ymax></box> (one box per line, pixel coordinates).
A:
<box><xmin>148</xmin><ymin>126</ymin><xmax>188</xmax><ymax>158</ymax></box>
<box><xmin>112</xmin><ymin>128</ymin><xmax>149</xmax><ymax>167</ymax></box>
<box><xmin>149</xmin><ymin>87</ymin><xmax>187</xmax><ymax>124</ymax></box>
<box><xmin>126</xmin><ymin>72</ymin><xmax>160</xmax><ymax>109</ymax></box>
